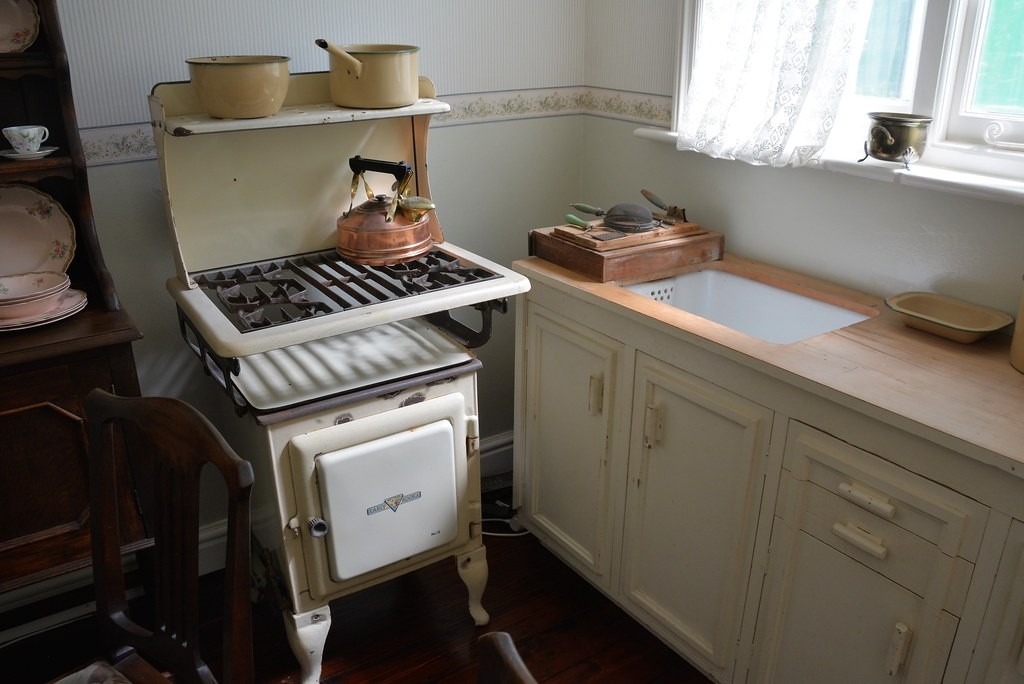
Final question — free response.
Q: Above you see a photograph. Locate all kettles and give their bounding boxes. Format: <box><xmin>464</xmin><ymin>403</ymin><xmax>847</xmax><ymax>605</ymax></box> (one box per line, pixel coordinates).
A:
<box><xmin>335</xmin><ymin>154</ymin><xmax>435</xmax><ymax>265</ymax></box>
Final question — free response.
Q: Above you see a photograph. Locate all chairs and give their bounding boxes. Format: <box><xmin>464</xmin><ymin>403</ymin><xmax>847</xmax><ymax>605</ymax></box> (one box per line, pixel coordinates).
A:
<box><xmin>50</xmin><ymin>388</ymin><xmax>258</xmax><ymax>684</ymax></box>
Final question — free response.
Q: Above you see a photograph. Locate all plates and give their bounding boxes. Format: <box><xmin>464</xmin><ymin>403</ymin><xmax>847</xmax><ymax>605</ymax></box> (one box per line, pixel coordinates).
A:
<box><xmin>0</xmin><ymin>271</ymin><xmax>88</xmax><ymax>332</ymax></box>
<box><xmin>0</xmin><ymin>146</ymin><xmax>58</xmax><ymax>160</ymax></box>
<box><xmin>0</xmin><ymin>186</ymin><xmax>76</xmax><ymax>273</ymax></box>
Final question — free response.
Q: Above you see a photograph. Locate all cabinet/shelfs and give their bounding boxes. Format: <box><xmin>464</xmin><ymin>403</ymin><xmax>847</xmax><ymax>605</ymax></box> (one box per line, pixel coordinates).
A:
<box><xmin>0</xmin><ymin>0</ymin><xmax>1024</xmax><ymax>684</ymax></box>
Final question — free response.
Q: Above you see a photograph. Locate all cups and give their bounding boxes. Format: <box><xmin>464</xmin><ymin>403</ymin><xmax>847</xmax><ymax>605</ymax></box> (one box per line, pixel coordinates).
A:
<box><xmin>2</xmin><ymin>125</ymin><xmax>49</xmax><ymax>153</ymax></box>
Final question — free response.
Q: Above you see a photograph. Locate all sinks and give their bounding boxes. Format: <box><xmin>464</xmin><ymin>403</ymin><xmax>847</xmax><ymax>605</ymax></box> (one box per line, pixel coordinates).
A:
<box><xmin>616</xmin><ymin>262</ymin><xmax>880</xmax><ymax>343</ymax></box>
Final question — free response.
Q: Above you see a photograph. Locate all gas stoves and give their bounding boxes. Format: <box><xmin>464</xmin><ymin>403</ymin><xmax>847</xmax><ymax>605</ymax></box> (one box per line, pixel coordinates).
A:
<box><xmin>164</xmin><ymin>241</ymin><xmax>535</xmax><ymax>409</ymax></box>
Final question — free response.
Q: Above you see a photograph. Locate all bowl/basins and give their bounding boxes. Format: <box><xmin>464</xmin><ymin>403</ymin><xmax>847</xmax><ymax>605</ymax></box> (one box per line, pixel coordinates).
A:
<box><xmin>885</xmin><ymin>290</ymin><xmax>1014</xmax><ymax>344</ymax></box>
<box><xmin>183</xmin><ymin>51</ymin><xmax>291</xmax><ymax>119</ymax></box>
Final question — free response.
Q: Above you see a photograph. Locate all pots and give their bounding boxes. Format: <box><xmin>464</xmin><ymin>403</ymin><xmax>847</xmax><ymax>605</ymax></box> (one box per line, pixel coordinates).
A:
<box><xmin>313</xmin><ymin>34</ymin><xmax>422</xmax><ymax>110</ymax></box>
